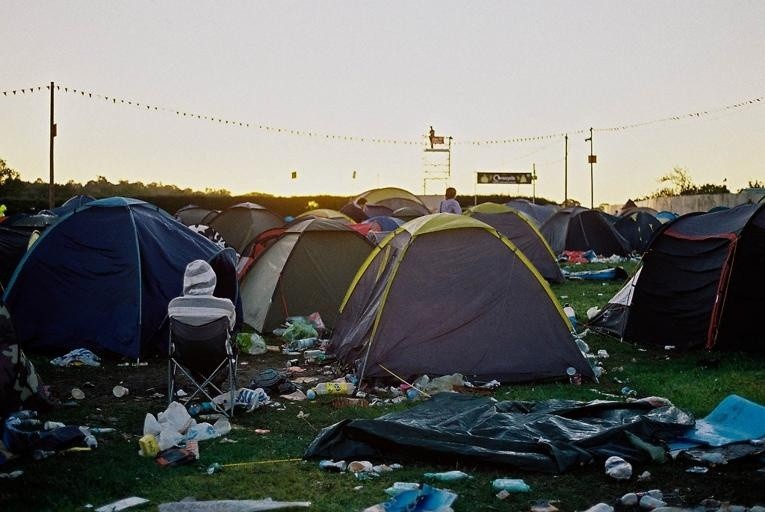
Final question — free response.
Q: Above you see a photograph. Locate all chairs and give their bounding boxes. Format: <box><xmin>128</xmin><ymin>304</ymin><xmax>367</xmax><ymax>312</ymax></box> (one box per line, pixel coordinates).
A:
<box><xmin>166</xmin><ymin>296</ymin><xmax>239</xmax><ymax>421</ymax></box>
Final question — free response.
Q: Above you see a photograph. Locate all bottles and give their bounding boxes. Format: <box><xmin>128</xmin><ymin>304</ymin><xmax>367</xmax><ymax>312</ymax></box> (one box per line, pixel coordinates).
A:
<box><xmin>306</xmin><ymin>388</ymin><xmax>316</xmax><ymax>400</ymax></box>
<box><xmin>20</xmin><ymin>419</ymin><xmax>40</xmax><ymax>426</ymax></box>
<box><xmin>10</xmin><ymin>409</ymin><xmax>37</xmax><ymax>419</ymax></box>
<box><xmin>563</xmin><ymin>302</ymin><xmax>577</xmax><ymax>333</ymax></box>
<box><xmin>604</xmin><ymin>455</ymin><xmax>633</xmax><ymax>481</ymax></box>
<box><xmin>44</xmin><ymin>421</ymin><xmax>66</xmax><ymax>431</ymax></box>
<box><xmin>289</xmin><ymin>338</ymin><xmax>314</xmax><ymax>349</ymax></box>
<box><xmin>304</xmin><ymin>350</ymin><xmax>326</xmax><ymax>360</ymax></box>
<box><xmin>639</xmin><ymin>496</ymin><xmax>668</xmax><ymax>511</ymax></box>
<box><xmin>287</xmin><ymin>359</ymin><xmax>299</xmax><ymax>366</ymax></box>
<box><xmin>83</xmin><ymin>434</ymin><xmax>99</xmax><ymax>450</ymax></box>
<box><xmin>616</xmin><ymin>489</ymin><xmax>663</xmax><ymax>506</ymax></box>
<box><xmin>583</xmin><ymin>502</ymin><xmax>615</xmax><ymax>512</ymax></box>
<box><xmin>188</xmin><ymin>403</ymin><xmax>201</xmax><ymax>415</ymax></box>
<box><xmin>314</xmin><ymin>382</ymin><xmax>357</xmax><ymax>396</ymax></box>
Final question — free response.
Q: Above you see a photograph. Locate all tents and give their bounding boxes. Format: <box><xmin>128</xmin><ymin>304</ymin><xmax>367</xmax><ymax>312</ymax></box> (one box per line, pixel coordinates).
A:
<box><xmin>1</xmin><ymin>188</ymin><xmax>764</xmax><ymax>396</ymax></box>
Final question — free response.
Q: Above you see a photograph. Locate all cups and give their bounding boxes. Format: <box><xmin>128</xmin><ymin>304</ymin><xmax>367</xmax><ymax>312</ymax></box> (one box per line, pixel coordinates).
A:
<box><xmin>347</xmin><ymin>459</ymin><xmax>373</xmax><ymax>473</ymax></box>
<box><xmin>113</xmin><ymin>386</ymin><xmax>130</xmax><ymax>398</ymax></box>
